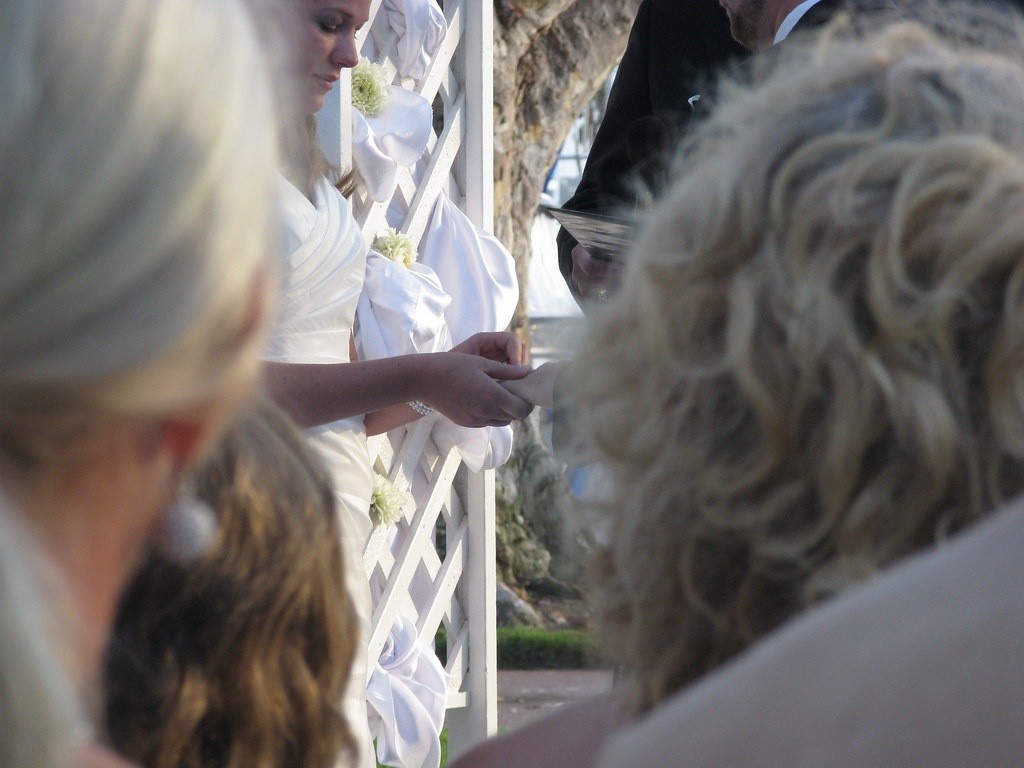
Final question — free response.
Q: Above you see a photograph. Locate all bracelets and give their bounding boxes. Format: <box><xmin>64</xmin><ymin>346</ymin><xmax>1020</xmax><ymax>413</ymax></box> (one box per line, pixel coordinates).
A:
<box><xmin>407</xmin><ymin>400</ymin><xmax>434</xmax><ymax>415</ymax></box>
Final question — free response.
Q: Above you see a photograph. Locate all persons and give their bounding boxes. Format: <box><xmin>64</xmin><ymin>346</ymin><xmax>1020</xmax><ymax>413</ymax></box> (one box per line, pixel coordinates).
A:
<box><xmin>0</xmin><ymin>0</ymin><xmax>531</xmax><ymax>768</ymax></box>
<box><xmin>450</xmin><ymin>0</ymin><xmax>1024</xmax><ymax>768</ymax></box>
